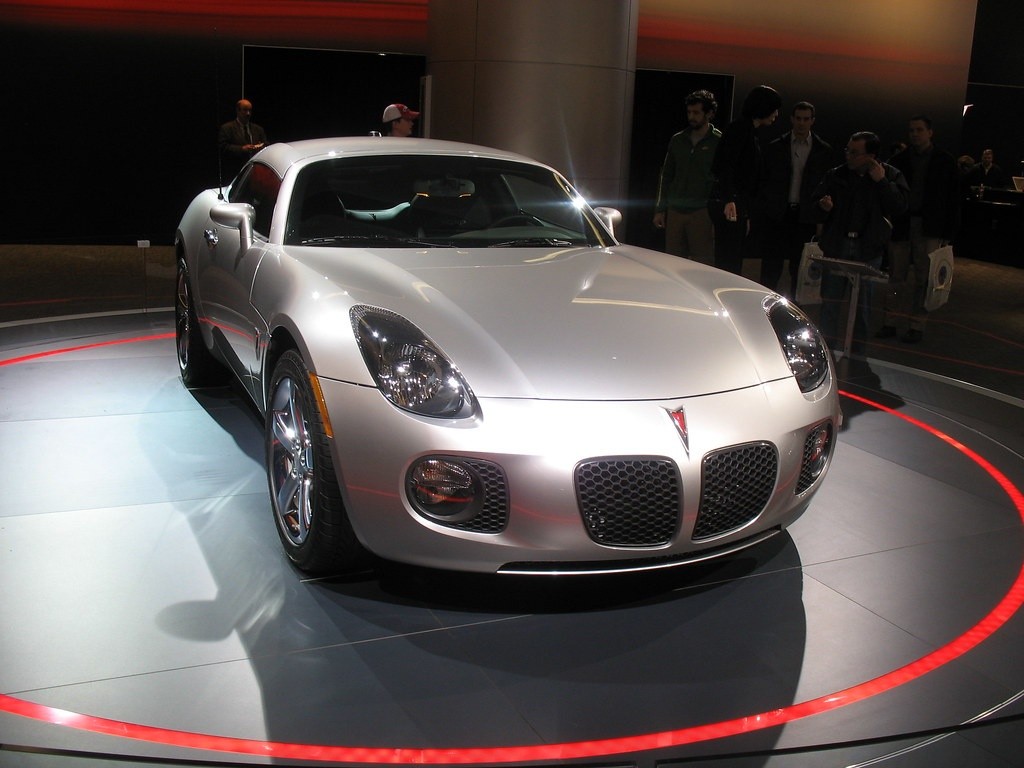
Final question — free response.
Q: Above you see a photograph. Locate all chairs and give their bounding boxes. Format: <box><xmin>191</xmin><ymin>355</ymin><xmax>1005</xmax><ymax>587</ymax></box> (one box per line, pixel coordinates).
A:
<box><xmin>250</xmin><ymin>192</ymin><xmax>431</xmax><ymax>238</ymax></box>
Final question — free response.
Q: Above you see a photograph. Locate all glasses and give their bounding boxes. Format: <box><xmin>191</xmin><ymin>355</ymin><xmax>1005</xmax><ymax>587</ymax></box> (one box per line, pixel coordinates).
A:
<box><xmin>844</xmin><ymin>146</ymin><xmax>869</xmax><ymax>158</ymax></box>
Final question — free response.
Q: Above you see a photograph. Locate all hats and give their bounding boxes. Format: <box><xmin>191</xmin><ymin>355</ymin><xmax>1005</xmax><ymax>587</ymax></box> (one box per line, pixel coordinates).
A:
<box><xmin>382</xmin><ymin>104</ymin><xmax>419</xmax><ymax>123</ymax></box>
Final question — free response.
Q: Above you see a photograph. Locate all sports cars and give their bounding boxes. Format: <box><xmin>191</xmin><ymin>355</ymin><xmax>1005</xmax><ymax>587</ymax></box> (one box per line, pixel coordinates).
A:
<box><xmin>174</xmin><ymin>138</ymin><xmax>842</xmax><ymax>580</ymax></box>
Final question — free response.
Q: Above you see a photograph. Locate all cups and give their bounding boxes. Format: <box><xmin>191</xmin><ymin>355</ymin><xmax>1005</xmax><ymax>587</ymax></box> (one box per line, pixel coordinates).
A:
<box><xmin>1012</xmin><ymin>176</ymin><xmax>1024</xmax><ymax>191</ymax></box>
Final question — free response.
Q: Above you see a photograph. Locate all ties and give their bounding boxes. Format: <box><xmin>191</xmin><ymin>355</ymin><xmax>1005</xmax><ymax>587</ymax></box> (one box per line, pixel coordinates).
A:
<box><xmin>243</xmin><ymin>126</ymin><xmax>251</xmax><ymax>144</ymax></box>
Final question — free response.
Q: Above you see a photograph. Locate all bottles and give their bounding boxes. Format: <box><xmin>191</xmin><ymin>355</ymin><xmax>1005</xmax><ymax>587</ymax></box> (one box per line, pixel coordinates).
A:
<box><xmin>970</xmin><ymin>185</ymin><xmax>992</xmax><ymax>201</ymax></box>
<box><xmin>796</xmin><ymin>241</ymin><xmax>823</xmax><ymax>305</ymax></box>
<box><xmin>776</xmin><ymin>259</ymin><xmax>792</xmax><ymax>300</ymax></box>
<box><xmin>844</xmin><ymin>231</ymin><xmax>863</xmax><ymax>262</ymax></box>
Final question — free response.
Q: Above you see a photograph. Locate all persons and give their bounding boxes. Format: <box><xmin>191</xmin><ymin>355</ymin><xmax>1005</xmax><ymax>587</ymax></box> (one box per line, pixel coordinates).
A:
<box><xmin>652</xmin><ymin>85</ymin><xmax>1008</xmax><ymax>372</ymax></box>
<box><xmin>380</xmin><ymin>102</ymin><xmax>421</xmax><ymax>138</ymax></box>
<box><xmin>218</xmin><ymin>98</ymin><xmax>271</xmax><ymax>174</ymax></box>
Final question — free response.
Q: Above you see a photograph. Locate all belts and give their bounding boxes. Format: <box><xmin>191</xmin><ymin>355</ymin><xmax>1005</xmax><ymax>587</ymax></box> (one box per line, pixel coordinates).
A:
<box><xmin>842</xmin><ymin>230</ymin><xmax>862</xmax><ymax>238</ymax></box>
<box><xmin>787</xmin><ymin>204</ymin><xmax>800</xmax><ymax>210</ymax></box>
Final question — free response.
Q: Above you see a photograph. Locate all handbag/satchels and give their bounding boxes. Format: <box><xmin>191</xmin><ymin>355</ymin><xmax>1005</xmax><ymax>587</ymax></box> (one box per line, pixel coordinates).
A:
<box><xmin>795</xmin><ymin>235</ymin><xmax>822</xmax><ymax>305</ymax></box>
<box><xmin>921</xmin><ymin>243</ymin><xmax>955</xmax><ymax>312</ymax></box>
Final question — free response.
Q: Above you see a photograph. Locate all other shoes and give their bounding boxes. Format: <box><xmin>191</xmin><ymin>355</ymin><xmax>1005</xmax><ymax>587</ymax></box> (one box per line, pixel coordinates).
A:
<box><xmin>874</xmin><ymin>326</ymin><xmax>897</xmax><ymax>338</ymax></box>
<box><xmin>901</xmin><ymin>327</ymin><xmax>923</xmax><ymax>344</ymax></box>
<box><xmin>855</xmin><ymin>333</ymin><xmax>869</xmax><ymax>355</ymax></box>
